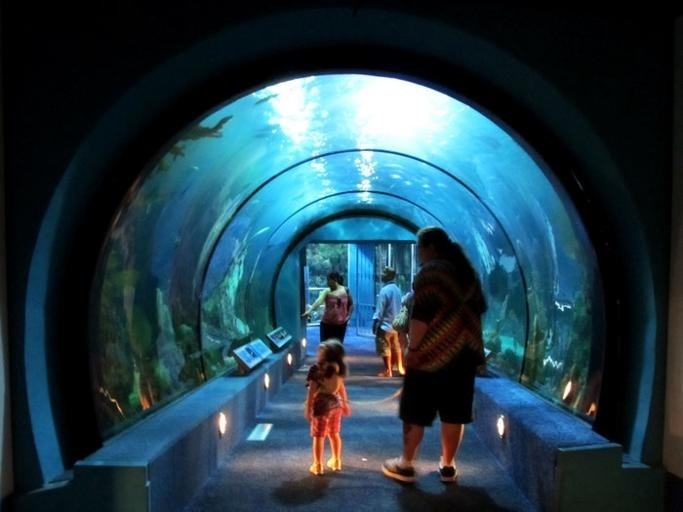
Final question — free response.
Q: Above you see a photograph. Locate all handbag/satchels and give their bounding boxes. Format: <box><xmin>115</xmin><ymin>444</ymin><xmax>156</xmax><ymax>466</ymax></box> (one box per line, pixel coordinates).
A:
<box><xmin>392</xmin><ymin>292</ymin><xmax>411</xmax><ymax>334</ymax></box>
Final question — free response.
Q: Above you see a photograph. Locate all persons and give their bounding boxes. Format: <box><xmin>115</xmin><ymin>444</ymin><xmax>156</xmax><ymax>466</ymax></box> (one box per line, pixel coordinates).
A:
<box><xmin>297</xmin><ymin>270</ymin><xmax>354</xmax><ymax>349</ymax></box>
<box><xmin>303</xmin><ymin>336</ymin><xmax>351</xmax><ymax>476</ymax></box>
<box><xmin>380</xmin><ymin>226</ymin><xmax>487</xmax><ymax>486</ymax></box>
<box><xmin>370</xmin><ymin>265</ymin><xmax>406</xmax><ymax>379</ymax></box>
<box><xmin>391</xmin><ymin>286</ymin><xmax>416</xmax><ymax>366</ymax></box>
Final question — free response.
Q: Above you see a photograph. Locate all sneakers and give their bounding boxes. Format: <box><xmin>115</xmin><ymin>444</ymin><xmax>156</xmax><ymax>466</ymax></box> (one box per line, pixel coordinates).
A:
<box><xmin>326</xmin><ymin>456</ymin><xmax>342</xmax><ymax>471</ymax></box>
<box><xmin>309</xmin><ymin>462</ymin><xmax>324</xmax><ymax>476</ymax></box>
<box><xmin>381</xmin><ymin>458</ymin><xmax>417</xmax><ymax>484</ymax></box>
<box><xmin>436</xmin><ymin>458</ymin><xmax>458</xmax><ymax>483</ymax></box>
<box><xmin>377</xmin><ymin>367</ymin><xmax>406</xmax><ymax>378</ymax></box>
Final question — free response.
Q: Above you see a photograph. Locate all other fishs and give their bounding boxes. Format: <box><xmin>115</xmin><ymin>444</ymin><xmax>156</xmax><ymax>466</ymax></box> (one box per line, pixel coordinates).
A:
<box><xmin>168</xmin><ymin>145</ymin><xmax>187</xmax><ymax>161</ymax></box>
<box><xmin>255</xmin><ymin>93</ymin><xmax>277</xmax><ymax>105</ymax></box>
<box><xmin>381</xmin><ymin>162</ymin><xmax>448</xmax><ymax>205</ymax></box>
